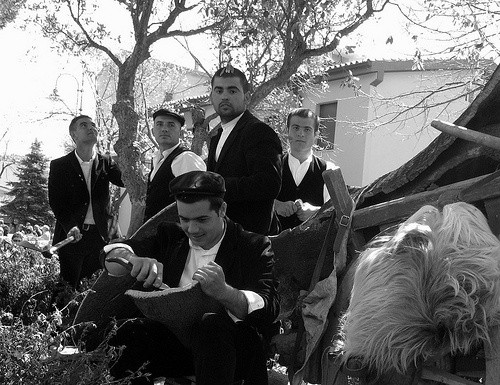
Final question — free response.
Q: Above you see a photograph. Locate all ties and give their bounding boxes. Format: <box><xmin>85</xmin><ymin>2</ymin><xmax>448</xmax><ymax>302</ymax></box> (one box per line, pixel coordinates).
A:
<box><xmin>154</xmin><ymin>155</ymin><xmax>164</xmax><ymax>169</ymax></box>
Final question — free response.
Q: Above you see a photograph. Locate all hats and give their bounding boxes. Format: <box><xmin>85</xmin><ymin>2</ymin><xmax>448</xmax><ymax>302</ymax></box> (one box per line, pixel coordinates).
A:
<box><xmin>153</xmin><ymin>109</ymin><xmax>185</xmax><ymax>127</ymax></box>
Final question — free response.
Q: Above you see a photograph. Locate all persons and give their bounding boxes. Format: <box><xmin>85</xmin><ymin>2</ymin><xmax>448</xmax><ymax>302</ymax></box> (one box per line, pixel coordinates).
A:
<box><xmin>336</xmin><ymin>202</ymin><xmax>500</xmax><ymax>385</ymax></box>
<box><xmin>46</xmin><ymin>115</ymin><xmax>126</xmax><ymax>325</ymax></box>
<box><xmin>268</xmin><ymin>108</ymin><xmax>340</xmax><ymax>230</ymax></box>
<box><xmin>96</xmin><ymin>171</ymin><xmax>280</xmax><ymax>385</ymax></box>
<box><xmin>207</xmin><ymin>66</ymin><xmax>283</xmax><ymax>236</ymax></box>
<box><xmin>143</xmin><ymin>109</ymin><xmax>208</xmax><ymax>226</ymax></box>
